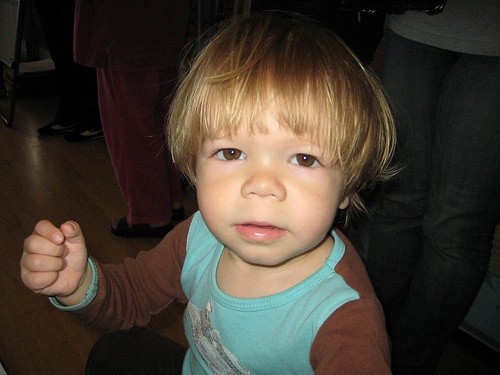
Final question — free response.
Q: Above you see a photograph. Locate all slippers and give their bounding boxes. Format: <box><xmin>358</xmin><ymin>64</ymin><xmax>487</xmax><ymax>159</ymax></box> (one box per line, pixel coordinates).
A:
<box><xmin>171</xmin><ymin>208</ymin><xmax>186</xmax><ymax>221</ymax></box>
<box><xmin>110</xmin><ymin>216</ymin><xmax>173</xmax><ymax>238</ymax></box>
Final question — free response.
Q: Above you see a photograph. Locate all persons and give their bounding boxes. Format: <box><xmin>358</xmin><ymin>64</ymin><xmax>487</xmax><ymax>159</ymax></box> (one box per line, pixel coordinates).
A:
<box><xmin>20</xmin><ymin>15</ymin><xmax>395</xmax><ymax>375</ymax></box>
<box><xmin>345</xmin><ymin>0</ymin><xmax>500</xmax><ymax>375</ymax></box>
<box><xmin>36</xmin><ymin>0</ymin><xmax>389</xmax><ymax>236</ymax></box>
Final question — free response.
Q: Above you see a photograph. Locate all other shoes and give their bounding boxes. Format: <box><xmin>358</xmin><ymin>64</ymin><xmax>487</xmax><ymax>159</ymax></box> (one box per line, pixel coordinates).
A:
<box><xmin>64</xmin><ymin>129</ymin><xmax>105</xmax><ymax>141</ymax></box>
<box><xmin>38</xmin><ymin>122</ymin><xmax>78</xmax><ymax>136</ymax></box>
